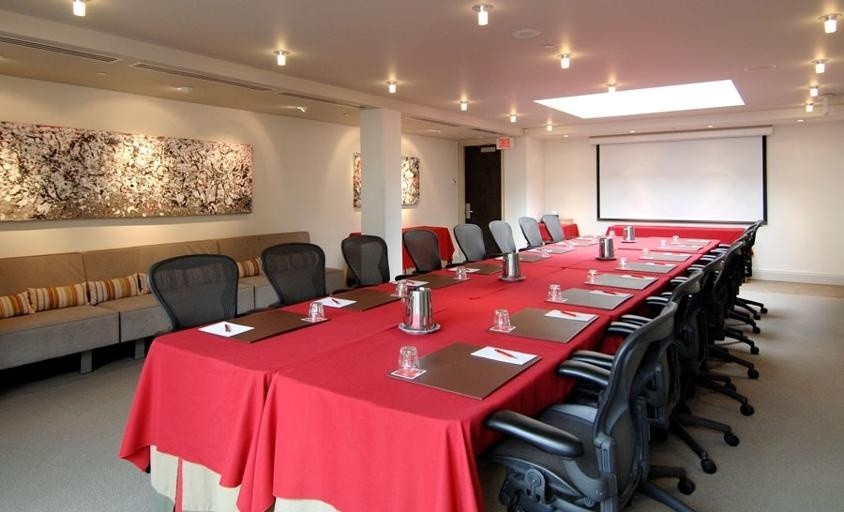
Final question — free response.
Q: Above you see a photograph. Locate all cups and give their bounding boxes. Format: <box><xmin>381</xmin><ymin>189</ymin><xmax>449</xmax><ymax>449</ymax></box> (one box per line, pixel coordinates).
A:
<box><xmin>395</xmin><ymin>280</ymin><xmax>409</xmax><ymax>299</ymax></box>
<box><xmin>540</xmin><ymin>231</ymin><xmax>682</xmax><ymax>305</ymax></box>
<box><xmin>396</xmin><ymin>344</ymin><xmax>421</xmax><ymax>378</ymax></box>
<box><xmin>456</xmin><ymin>265</ymin><xmax>469</xmax><ymax>282</ymax></box>
<box><xmin>491</xmin><ymin>308</ymin><xmax>514</xmax><ymax>332</ymax></box>
<box><xmin>401</xmin><ymin>289</ymin><xmax>435</xmax><ymax>329</ymax></box>
<box><xmin>503</xmin><ymin>251</ymin><xmax>520</xmax><ymax>279</ymax></box>
<box><xmin>306</xmin><ymin>300</ymin><xmax>326</xmax><ymax>324</ymax></box>
<box><xmin>623</xmin><ymin>226</ymin><xmax>635</xmax><ymax>242</ymax></box>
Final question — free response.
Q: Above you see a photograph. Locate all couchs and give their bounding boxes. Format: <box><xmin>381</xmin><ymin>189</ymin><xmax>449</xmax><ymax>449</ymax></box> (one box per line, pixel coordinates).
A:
<box><xmin>82</xmin><ymin>239</ymin><xmax>255</xmax><ymax>359</ymax></box>
<box><xmin>1</xmin><ymin>252</ymin><xmax>120</xmax><ymax>375</ymax></box>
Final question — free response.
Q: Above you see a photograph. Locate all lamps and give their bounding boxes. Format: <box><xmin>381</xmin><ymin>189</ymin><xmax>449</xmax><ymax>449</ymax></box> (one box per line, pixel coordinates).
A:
<box><xmin>472</xmin><ymin>3</ymin><xmax>494</xmax><ymax>26</ymax></box>
<box><xmin>509</xmin><ymin>113</ymin><xmax>517</xmax><ymax>124</ymax></box>
<box><xmin>803</xmin><ymin>13</ymin><xmax>840</xmax><ymax>113</ymax></box>
<box><xmin>273</xmin><ymin>50</ymin><xmax>290</xmax><ymax>66</ymax></box>
<box><xmin>73</xmin><ymin>0</ymin><xmax>86</xmax><ymax>16</ymax></box>
<box><xmin>460</xmin><ymin>100</ymin><xmax>468</xmax><ymax>112</ymax></box>
<box><xmin>555</xmin><ymin>52</ymin><xmax>572</xmax><ymax>69</ymax></box>
<box><xmin>547</xmin><ymin>124</ymin><xmax>553</xmax><ymax>132</ymax></box>
<box><xmin>607</xmin><ymin>81</ymin><xmax>616</xmax><ymax>93</ymax></box>
<box><xmin>385</xmin><ymin>81</ymin><xmax>397</xmax><ymax>94</ymax></box>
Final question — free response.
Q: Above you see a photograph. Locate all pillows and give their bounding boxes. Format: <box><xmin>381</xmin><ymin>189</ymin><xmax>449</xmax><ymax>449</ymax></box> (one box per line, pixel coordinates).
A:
<box><xmin>0</xmin><ymin>290</ymin><xmax>35</xmax><ymax>319</ymax></box>
<box><xmin>27</xmin><ymin>281</ymin><xmax>89</xmax><ymax>312</ymax></box>
<box><xmin>88</xmin><ymin>273</ymin><xmax>140</xmax><ymax>306</ymax></box>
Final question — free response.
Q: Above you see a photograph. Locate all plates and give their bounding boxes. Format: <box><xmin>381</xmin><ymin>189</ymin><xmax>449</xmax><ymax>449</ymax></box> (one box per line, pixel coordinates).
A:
<box><xmin>397</xmin><ymin>323</ymin><xmax>441</xmax><ymax>335</ymax></box>
<box><xmin>499</xmin><ymin>276</ymin><xmax>527</xmax><ymax>284</ymax></box>
